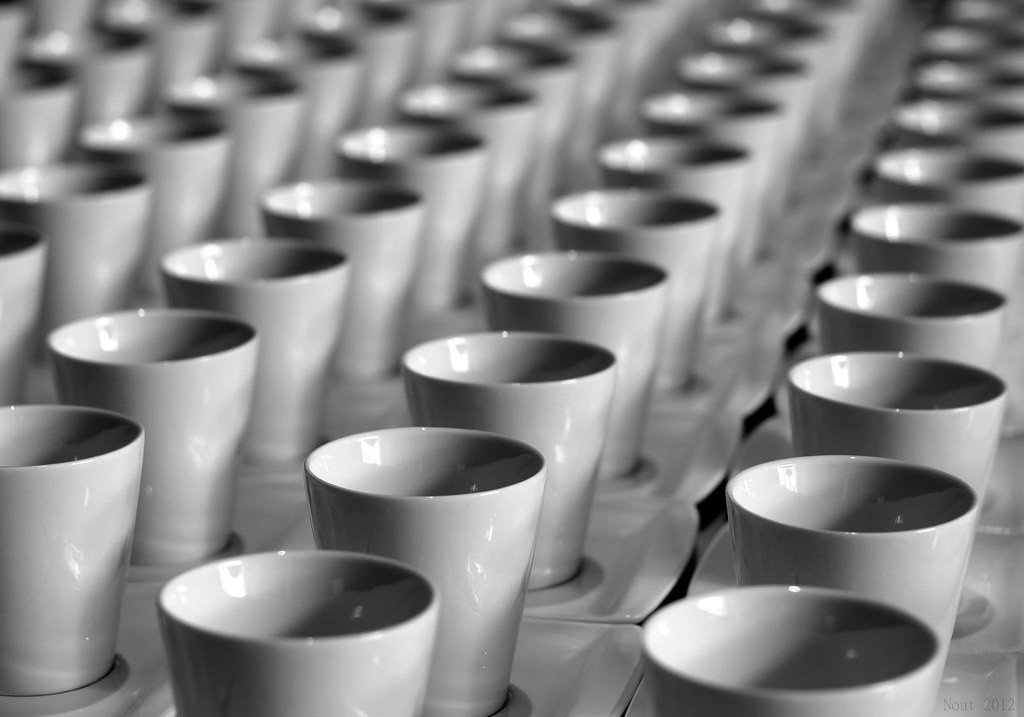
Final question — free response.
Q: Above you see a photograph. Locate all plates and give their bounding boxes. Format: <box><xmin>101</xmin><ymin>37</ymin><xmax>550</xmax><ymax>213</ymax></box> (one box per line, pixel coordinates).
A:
<box><xmin>0</xmin><ymin>0</ymin><xmax>1024</xmax><ymax>717</ymax></box>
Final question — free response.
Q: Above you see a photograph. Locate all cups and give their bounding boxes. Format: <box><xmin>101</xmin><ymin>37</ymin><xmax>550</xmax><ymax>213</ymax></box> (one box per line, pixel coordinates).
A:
<box><xmin>787</xmin><ymin>353</ymin><xmax>1007</xmax><ymax>510</ymax></box>
<box><xmin>401</xmin><ymin>335</ymin><xmax>616</xmax><ymax>592</ymax></box>
<box><xmin>248</xmin><ymin>31</ymin><xmax>365</xmax><ymax>189</ymax></box>
<box><xmin>164</xmin><ymin>67</ymin><xmax>305</xmax><ymax>239</ymax></box>
<box><xmin>80</xmin><ymin>25</ymin><xmax>153</xmax><ymax>113</ymax></box>
<box><xmin>450</xmin><ymin>42</ymin><xmax>575</xmax><ymax>186</ymax></box>
<box><xmin>481</xmin><ymin>252</ymin><xmax>665</xmax><ymax>480</ymax></box>
<box><xmin>153</xmin><ymin>0</ymin><xmax>221</xmax><ymax>101</ymax></box>
<box><xmin>676</xmin><ymin>51</ymin><xmax>808</xmax><ymax>208</ymax></box>
<box><xmin>47</xmin><ymin>309</ymin><xmax>260</xmax><ymax>566</ymax></box>
<box><xmin>0</xmin><ymin>58</ymin><xmax>80</xmax><ymax>167</ymax></box>
<box><xmin>641</xmin><ymin>92</ymin><xmax>783</xmax><ymax>262</ymax></box>
<box><xmin>916</xmin><ymin>18</ymin><xmax>1024</xmax><ymax>55</ymax></box>
<box><xmin>164</xmin><ymin>241</ymin><xmax>351</xmax><ymax>473</ymax></box>
<box><xmin>308</xmin><ymin>3</ymin><xmax>412</xmax><ymax>120</ymax></box>
<box><xmin>0</xmin><ymin>227</ymin><xmax>44</xmax><ymax>404</ymax></box>
<box><xmin>896</xmin><ymin>98</ymin><xmax>1024</xmax><ymax>143</ymax></box>
<box><xmin>342</xmin><ymin>126</ymin><xmax>485</xmax><ymax>317</ymax></box>
<box><xmin>816</xmin><ymin>275</ymin><xmax>1007</xmax><ymax>392</ymax></box>
<box><xmin>80</xmin><ymin>113</ymin><xmax>232</xmax><ymax>248</ymax></box>
<box><xmin>907</xmin><ymin>57</ymin><xmax>1024</xmax><ymax>100</ymax></box>
<box><xmin>852</xmin><ymin>204</ymin><xmax>1024</xmax><ymax>276</ymax></box>
<box><xmin>502</xmin><ymin>8</ymin><xmax>617</xmax><ymax>144</ymax></box>
<box><xmin>643</xmin><ymin>587</ymin><xmax>944</xmax><ymax>717</ymax></box>
<box><xmin>397</xmin><ymin>78</ymin><xmax>538</xmax><ymax>241</ymax></box>
<box><xmin>159</xmin><ymin>552</ymin><xmax>440</xmax><ymax>717</ymax></box>
<box><xmin>0</xmin><ymin>168</ymin><xmax>150</xmax><ymax>310</ymax></box>
<box><xmin>878</xmin><ymin>144</ymin><xmax>1024</xmax><ymax>203</ymax></box>
<box><xmin>0</xmin><ymin>404</ymin><xmax>144</xmax><ymax>697</ymax></box>
<box><xmin>949</xmin><ymin>0</ymin><xmax>1024</xmax><ymax>27</ymax></box>
<box><xmin>304</xmin><ymin>428</ymin><xmax>546</xmax><ymax>717</ymax></box>
<box><xmin>551</xmin><ymin>189</ymin><xmax>722</xmax><ymax>394</ymax></box>
<box><xmin>727</xmin><ymin>454</ymin><xmax>978</xmax><ymax>665</ymax></box>
<box><xmin>705</xmin><ymin>13</ymin><xmax>829</xmax><ymax>165</ymax></box>
<box><xmin>597</xmin><ymin>136</ymin><xmax>748</xmax><ymax>338</ymax></box>
<box><xmin>263</xmin><ymin>182</ymin><xmax>428</xmax><ymax>376</ymax></box>
